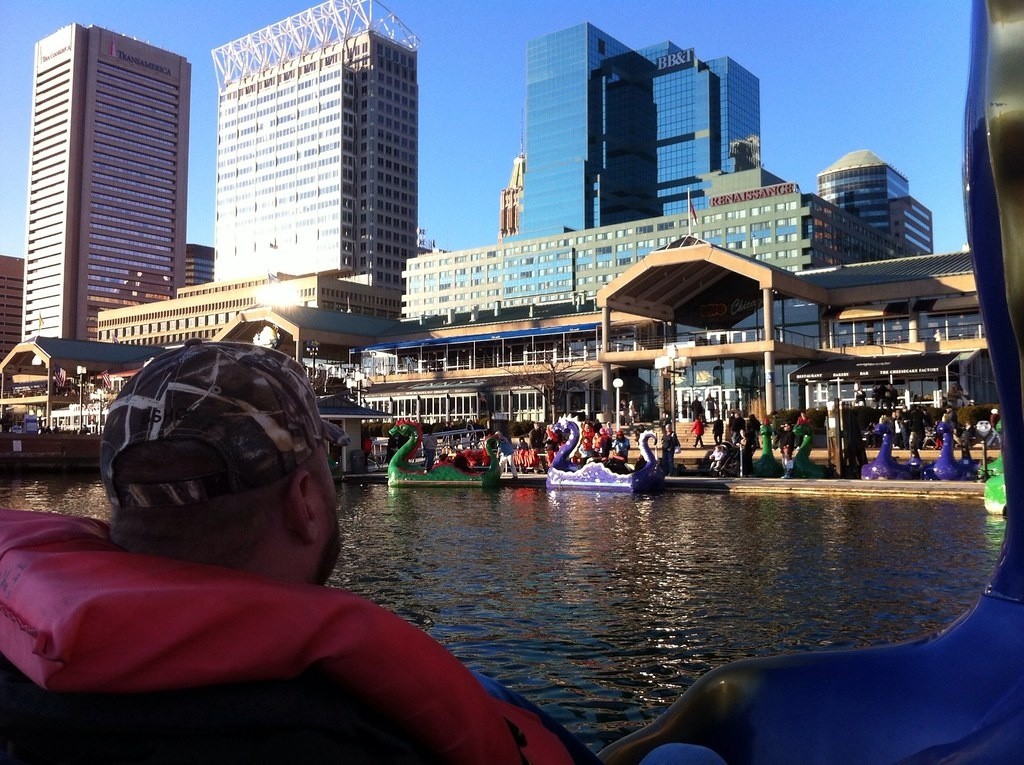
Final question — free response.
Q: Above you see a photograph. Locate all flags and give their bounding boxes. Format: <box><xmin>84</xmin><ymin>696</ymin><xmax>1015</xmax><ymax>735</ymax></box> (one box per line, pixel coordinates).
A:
<box><xmin>101</xmin><ymin>368</ymin><xmax>113</xmax><ymax>389</ymax></box>
<box><xmin>689</xmin><ymin>198</ymin><xmax>699</xmax><ymax>225</ymax></box>
<box><xmin>39</xmin><ymin>315</ymin><xmax>44</xmax><ymax>330</ymax></box>
<box><xmin>53</xmin><ymin>364</ymin><xmax>67</xmax><ymax>388</ymax></box>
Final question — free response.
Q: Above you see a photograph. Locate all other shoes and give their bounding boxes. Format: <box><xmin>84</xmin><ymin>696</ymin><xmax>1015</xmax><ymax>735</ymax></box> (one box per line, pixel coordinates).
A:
<box><xmin>693</xmin><ymin>445</ymin><xmax>696</xmax><ymax>448</ymax></box>
<box><xmin>700</xmin><ymin>446</ymin><xmax>704</xmax><ymax>448</ymax></box>
<box><xmin>533</xmin><ymin>469</ymin><xmax>540</xmax><ymax>474</ymax></box>
<box><xmin>781</xmin><ymin>474</ymin><xmax>794</xmax><ymax>479</ymax></box>
<box><xmin>542</xmin><ymin>469</ymin><xmax>547</xmax><ymax>474</ymax></box>
<box><xmin>510</xmin><ymin>476</ymin><xmax>518</xmax><ymax>479</ymax></box>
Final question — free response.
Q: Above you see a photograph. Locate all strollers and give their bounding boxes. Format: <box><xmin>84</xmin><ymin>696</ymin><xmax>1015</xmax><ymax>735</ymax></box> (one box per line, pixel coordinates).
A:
<box><xmin>709</xmin><ymin>441</ymin><xmax>740</xmax><ymax>477</ymax></box>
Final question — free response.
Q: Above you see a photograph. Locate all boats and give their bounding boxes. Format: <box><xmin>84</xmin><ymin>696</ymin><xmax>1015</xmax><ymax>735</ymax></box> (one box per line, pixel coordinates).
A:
<box><xmin>984</xmin><ymin>419</ymin><xmax>1009</xmax><ymax>516</ymax></box>
<box><xmin>860</xmin><ymin>414</ymin><xmax>920</xmax><ymax>482</ymax></box>
<box><xmin>788</xmin><ymin>413</ymin><xmax>832</xmax><ymax>479</ymax></box>
<box><xmin>751</xmin><ymin>416</ymin><xmax>784</xmax><ymax>478</ymax></box>
<box><xmin>545</xmin><ymin>414</ymin><xmax>665</xmax><ymax>495</ymax></box>
<box><xmin>921</xmin><ymin>410</ymin><xmax>977</xmax><ymax>481</ymax></box>
<box><xmin>387</xmin><ymin>420</ymin><xmax>515</xmax><ymax>490</ymax></box>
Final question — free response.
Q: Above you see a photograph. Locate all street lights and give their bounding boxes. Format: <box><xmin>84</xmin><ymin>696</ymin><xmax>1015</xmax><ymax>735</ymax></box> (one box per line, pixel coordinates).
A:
<box><xmin>77</xmin><ymin>365</ymin><xmax>87</xmax><ymax>433</ymax></box>
<box><xmin>307</xmin><ymin>347</ymin><xmax>319</xmax><ymax>386</ymax></box>
<box><xmin>612</xmin><ymin>377</ymin><xmax>625</xmax><ymax>434</ymax></box>
<box><xmin>655</xmin><ymin>344</ymin><xmax>692</xmax><ymax>438</ymax></box>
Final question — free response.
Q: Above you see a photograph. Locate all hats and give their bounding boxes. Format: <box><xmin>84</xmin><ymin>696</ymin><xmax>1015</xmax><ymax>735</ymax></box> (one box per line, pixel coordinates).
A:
<box><xmin>616</xmin><ymin>431</ymin><xmax>623</xmax><ymax>436</ymax></box>
<box><xmin>99</xmin><ymin>335</ymin><xmax>352</xmax><ymax>508</ymax></box>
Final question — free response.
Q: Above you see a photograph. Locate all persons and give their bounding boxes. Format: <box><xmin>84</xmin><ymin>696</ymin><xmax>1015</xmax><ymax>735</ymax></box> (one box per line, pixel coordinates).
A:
<box><xmin>361</xmin><ymin>378</ymin><xmax>993</xmax><ymax>472</ymax></box>
<box><xmin>0</xmin><ymin>336</ymin><xmax>727</xmax><ymax>765</ymax></box>
